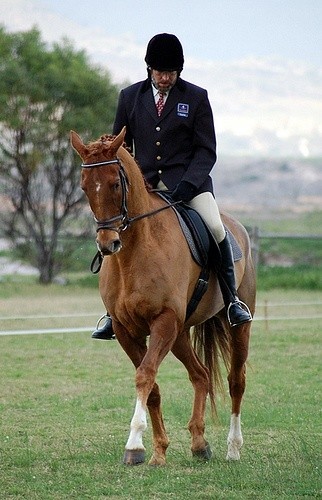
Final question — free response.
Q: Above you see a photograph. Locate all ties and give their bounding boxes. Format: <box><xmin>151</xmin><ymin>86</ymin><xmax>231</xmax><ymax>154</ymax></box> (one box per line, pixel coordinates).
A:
<box><xmin>157</xmin><ymin>90</ymin><xmax>166</xmax><ymax>115</ymax></box>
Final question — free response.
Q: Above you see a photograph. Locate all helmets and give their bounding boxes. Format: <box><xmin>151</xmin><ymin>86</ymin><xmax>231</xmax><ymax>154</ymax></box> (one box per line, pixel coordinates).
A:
<box><xmin>145</xmin><ymin>32</ymin><xmax>185</xmax><ymax>71</ymax></box>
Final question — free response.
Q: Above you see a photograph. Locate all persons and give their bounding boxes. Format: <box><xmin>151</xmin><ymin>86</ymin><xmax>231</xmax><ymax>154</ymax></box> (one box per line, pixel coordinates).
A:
<box><xmin>92</xmin><ymin>33</ymin><xmax>251</xmax><ymax>340</ymax></box>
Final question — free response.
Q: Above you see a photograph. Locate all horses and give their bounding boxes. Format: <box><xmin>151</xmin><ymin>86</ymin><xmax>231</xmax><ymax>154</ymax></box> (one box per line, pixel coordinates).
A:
<box><xmin>70</xmin><ymin>125</ymin><xmax>257</xmax><ymax>466</ymax></box>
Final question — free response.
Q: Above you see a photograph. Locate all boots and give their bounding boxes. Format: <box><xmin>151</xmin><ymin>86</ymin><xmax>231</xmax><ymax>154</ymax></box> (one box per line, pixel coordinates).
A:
<box><xmin>91</xmin><ymin>313</ymin><xmax>115</xmax><ymax>340</ymax></box>
<box><xmin>218</xmin><ymin>232</ymin><xmax>254</xmax><ymax>327</ymax></box>
